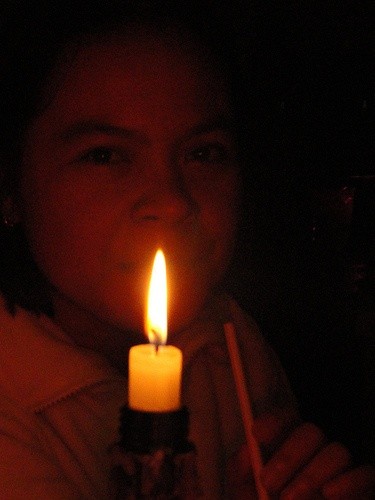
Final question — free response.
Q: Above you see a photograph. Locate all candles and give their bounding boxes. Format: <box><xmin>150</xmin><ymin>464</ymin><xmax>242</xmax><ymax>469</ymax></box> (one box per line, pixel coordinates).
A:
<box><xmin>128</xmin><ymin>345</ymin><xmax>184</xmax><ymax>412</ymax></box>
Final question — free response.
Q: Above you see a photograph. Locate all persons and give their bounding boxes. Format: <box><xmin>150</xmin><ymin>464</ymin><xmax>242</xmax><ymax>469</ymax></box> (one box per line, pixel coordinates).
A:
<box><xmin>0</xmin><ymin>12</ymin><xmax>375</xmax><ymax>500</ymax></box>
